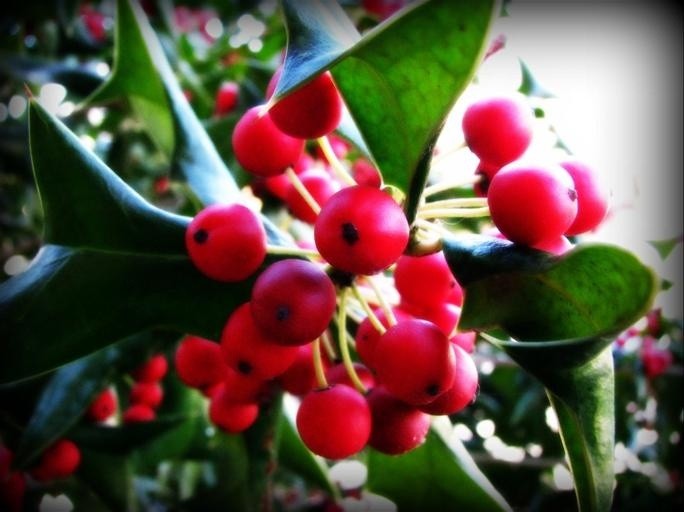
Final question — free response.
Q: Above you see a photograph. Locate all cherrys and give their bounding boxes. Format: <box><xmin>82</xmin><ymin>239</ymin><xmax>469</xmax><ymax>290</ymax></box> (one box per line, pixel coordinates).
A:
<box><xmin>0</xmin><ymin>0</ymin><xmax>673</xmax><ymax>512</ymax></box>
